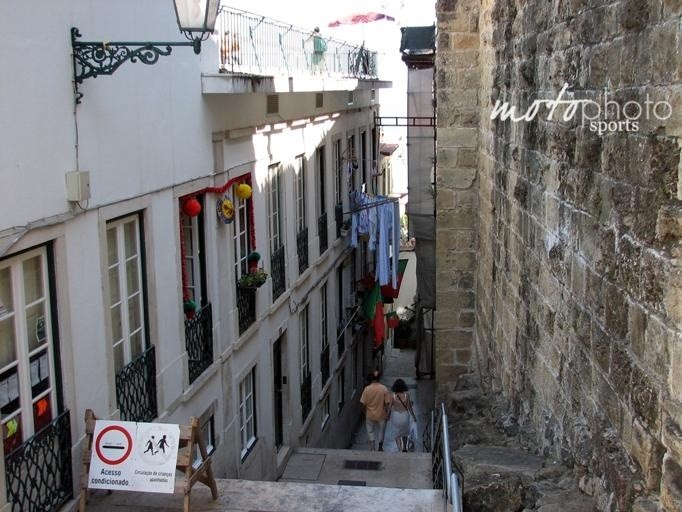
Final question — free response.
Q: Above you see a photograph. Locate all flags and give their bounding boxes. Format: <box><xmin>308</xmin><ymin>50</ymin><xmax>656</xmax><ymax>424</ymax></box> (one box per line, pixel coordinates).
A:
<box><xmin>361</xmin><ymin>256</ymin><xmax>409</xmax><ymax>348</ymax></box>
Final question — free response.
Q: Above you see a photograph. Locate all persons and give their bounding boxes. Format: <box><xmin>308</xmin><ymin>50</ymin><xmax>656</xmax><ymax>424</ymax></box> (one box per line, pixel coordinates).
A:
<box><xmin>360</xmin><ymin>370</ymin><xmax>390</xmax><ymax>452</ymax></box>
<box><xmin>386</xmin><ymin>379</ymin><xmax>417</xmax><ymax>453</ymax></box>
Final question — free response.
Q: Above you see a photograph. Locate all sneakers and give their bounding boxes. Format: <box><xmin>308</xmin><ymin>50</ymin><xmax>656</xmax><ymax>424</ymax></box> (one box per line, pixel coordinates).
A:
<box><xmin>402</xmin><ymin>449</ymin><xmax>408</xmax><ymax>452</ymax></box>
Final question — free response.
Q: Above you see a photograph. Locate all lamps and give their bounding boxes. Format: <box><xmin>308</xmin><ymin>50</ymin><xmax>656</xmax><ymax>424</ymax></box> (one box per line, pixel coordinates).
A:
<box><xmin>69</xmin><ymin>1</ymin><xmax>221</xmax><ymax>106</ymax></box>
